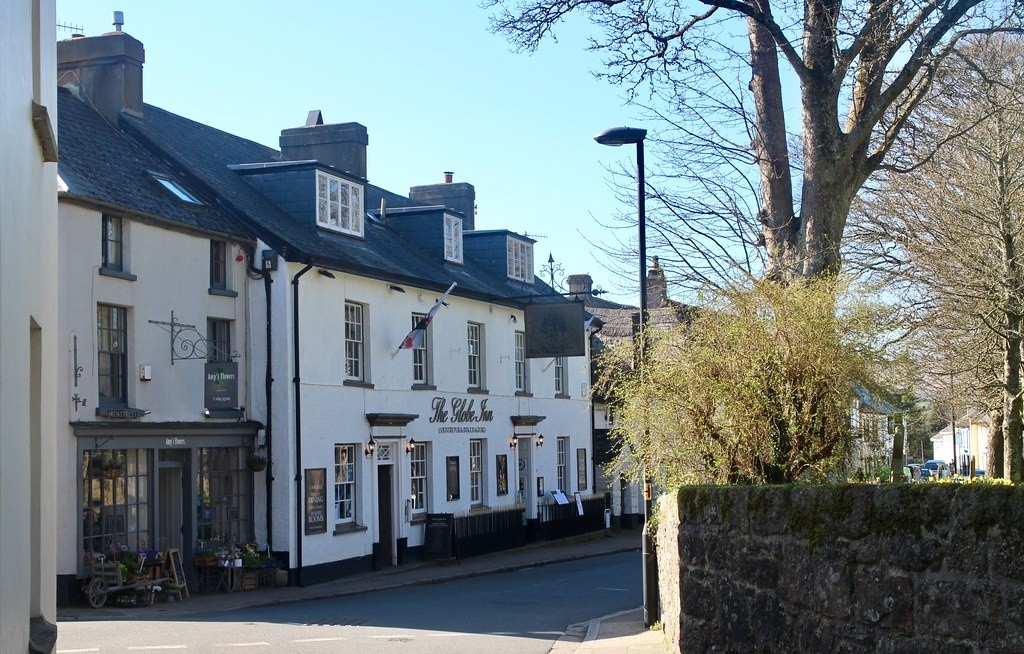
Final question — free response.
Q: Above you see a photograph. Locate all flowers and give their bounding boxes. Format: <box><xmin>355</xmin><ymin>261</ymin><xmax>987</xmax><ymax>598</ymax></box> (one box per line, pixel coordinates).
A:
<box><xmin>101</xmin><ymin>459</ymin><xmax>125</xmax><ymax>471</ymax></box>
<box><xmin>248</xmin><ymin>455</ymin><xmax>266</xmax><ymax>464</ymax></box>
<box><xmin>195</xmin><ymin>535</ymin><xmax>258</xmax><ymax>558</ymax></box>
<box><xmin>135</xmin><ymin>549</ymin><xmax>159</xmax><ymax>560</ymax></box>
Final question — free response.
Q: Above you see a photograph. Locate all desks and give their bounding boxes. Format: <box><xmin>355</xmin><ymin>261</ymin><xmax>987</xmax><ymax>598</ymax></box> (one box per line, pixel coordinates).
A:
<box><xmin>213</xmin><ymin>565</ymin><xmax>247</xmax><ymax>594</ymax></box>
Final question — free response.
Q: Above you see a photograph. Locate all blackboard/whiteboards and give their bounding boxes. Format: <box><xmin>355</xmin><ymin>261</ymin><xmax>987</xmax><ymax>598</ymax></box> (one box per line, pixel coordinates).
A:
<box><xmin>423</xmin><ymin>512</ymin><xmax>454</xmax><ymax>559</ymax></box>
<box><xmin>168</xmin><ymin>548</ymin><xmax>186</xmax><ymax>588</ymax></box>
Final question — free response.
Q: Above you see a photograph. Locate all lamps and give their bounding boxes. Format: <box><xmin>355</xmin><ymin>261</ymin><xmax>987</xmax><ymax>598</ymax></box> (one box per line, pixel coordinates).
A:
<box><xmin>510</xmin><ymin>432</ymin><xmax>518</xmax><ymax>448</ymax></box>
<box><xmin>365</xmin><ymin>435</ymin><xmax>376</xmax><ymax>456</ymax></box>
<box><xmin>406</xmin><ymin>437</ymin><xmax>415</xmax><ymax>454</ymax></box>
<box><xmin>536</xmin><ymin>434</ymin><xmax>544</xmax><ymax>447</ymax></box>
<box><xmin>318</xmin><ymin>270</ymin><xmax>335</xmax><ymax>279</ymax></box>
<box><xmin>436</xmin><ymin>299</ymin><xmax>450</xmax><ymax>309</ymax></box>
<box><xmin>511</xmin><ymin>315</ymin><xmax>518</xmax><ymax>323</ymax></box>
<box><xmin>390</xmin><ymin>285</ymin><xmax>405</xmax><ymax>293</ymax></box>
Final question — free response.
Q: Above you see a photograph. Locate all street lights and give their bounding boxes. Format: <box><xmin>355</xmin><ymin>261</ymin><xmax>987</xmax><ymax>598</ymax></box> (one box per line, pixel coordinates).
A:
<box><xmin>593</xmin><ymin>125</ymin><xmax>664</xmax><ymax>629</ymax></box>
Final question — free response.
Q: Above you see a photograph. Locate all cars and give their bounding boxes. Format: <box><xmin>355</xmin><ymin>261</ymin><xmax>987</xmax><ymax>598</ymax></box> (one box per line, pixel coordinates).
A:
<box><xmin>974</xmin><ymin>469</ymin><xmax>987</xmax><ymax>479</ymax></box>
<box><xmin>922</xmin><ymin>459</ymin><xmax>946</xmax><ymax>477</ymax></box>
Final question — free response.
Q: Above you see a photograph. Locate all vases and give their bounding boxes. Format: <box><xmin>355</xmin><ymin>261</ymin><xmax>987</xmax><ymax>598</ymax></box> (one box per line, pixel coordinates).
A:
<box><xmin>235</xmin><ymin>559</ymin><xmax>242</xmax><ymax>567</ymax></box>
